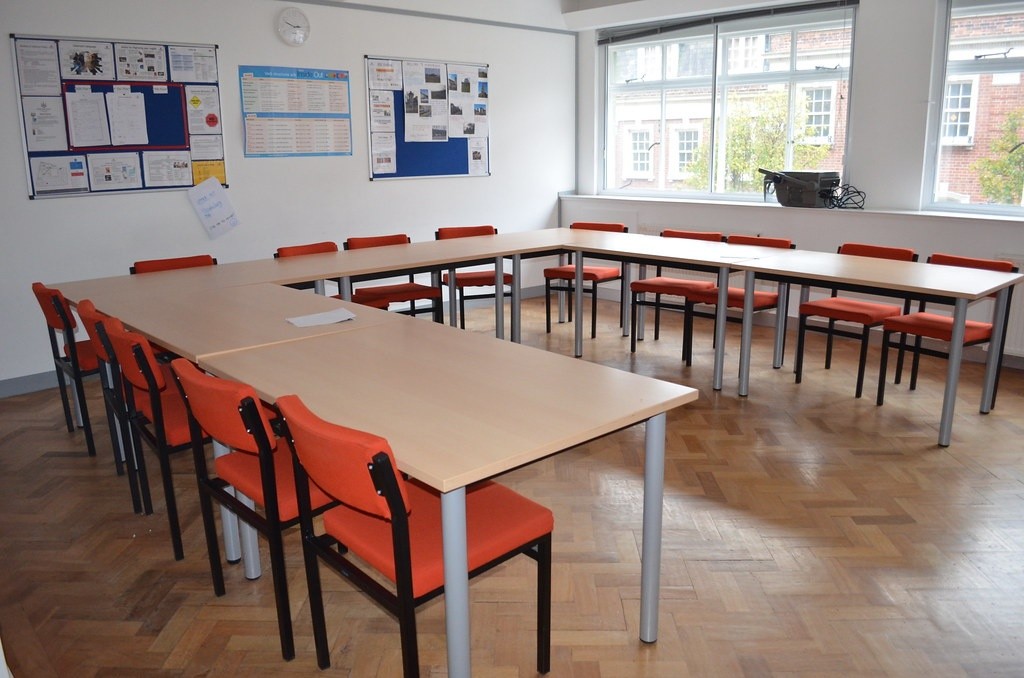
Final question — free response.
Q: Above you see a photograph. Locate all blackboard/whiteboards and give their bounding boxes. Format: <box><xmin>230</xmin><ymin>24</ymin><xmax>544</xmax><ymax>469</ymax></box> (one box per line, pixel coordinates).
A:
<box><xmin>364</xmin><ymin>55</ymin><xmax>491</xmax><ymax>181</ymax></box>
<box><xmin>9</xmin><ymin>33</ymin><xmax>229</xmax><ymax>200</ymax></box>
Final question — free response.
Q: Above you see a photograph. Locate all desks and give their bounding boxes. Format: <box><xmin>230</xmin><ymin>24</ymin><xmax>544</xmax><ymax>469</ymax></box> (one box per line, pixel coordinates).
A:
<box><xmin>43</xmin><ymin>227</ymin><xmax>1024</xmax><ymax>678</ymax></box>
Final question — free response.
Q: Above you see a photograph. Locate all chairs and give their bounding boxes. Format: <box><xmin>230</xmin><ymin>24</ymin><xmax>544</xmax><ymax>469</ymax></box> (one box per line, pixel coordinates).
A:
<box><xmin>32</xmin><ymin>221</ymin><xmax>1020</xmax><ymax>678</ymax></box>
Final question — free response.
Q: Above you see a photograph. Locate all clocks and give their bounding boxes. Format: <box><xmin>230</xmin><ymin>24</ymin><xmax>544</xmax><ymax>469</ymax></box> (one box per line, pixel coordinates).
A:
<box><xmin>277</xmin><ymin>7</ymin><xmax>310</xmax><ymax>47</ymax></box>
